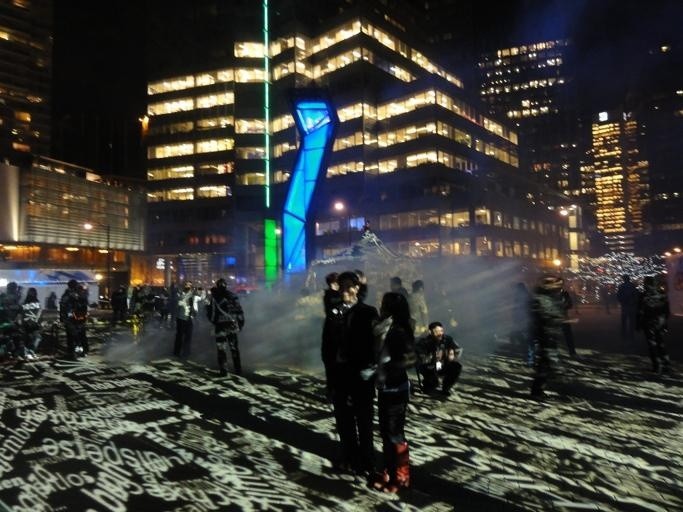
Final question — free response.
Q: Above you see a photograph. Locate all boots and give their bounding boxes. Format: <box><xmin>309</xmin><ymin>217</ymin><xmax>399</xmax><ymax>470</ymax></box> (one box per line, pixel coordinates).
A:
<box><xmin>395</xmin><ymin>441</ymin><xmax>410</xmax><ymax>487</ymax></box>
<box><xmin>382</xmin><ymin>446</ymin><xmax>393</xmax><ymax>485</ymax></box>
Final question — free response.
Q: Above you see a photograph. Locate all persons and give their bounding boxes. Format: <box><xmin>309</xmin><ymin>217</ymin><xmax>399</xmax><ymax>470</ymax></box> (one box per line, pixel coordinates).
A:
<box><xmin>108</xmin><ymin>277</ymin><xmax>246</xmax><ymax>377</ymax></box>
<box><xmin>508</xmin><ymin>271</ymin><xmax>581</xmax><ymax>402</ymax></box>
<box><xmin>1</xmin><ymin>281</ymin><xmax>88</xmax><ymax>359</ymax></box>
<box><xmin>321</xmin><ymin>270</ymin><xmax>463</xmax><ymax>494</ymax></box>
<box><xmin>616</xmin><ymin>272</ymin><xmax>674</xmax><ymax>372</ymax></box>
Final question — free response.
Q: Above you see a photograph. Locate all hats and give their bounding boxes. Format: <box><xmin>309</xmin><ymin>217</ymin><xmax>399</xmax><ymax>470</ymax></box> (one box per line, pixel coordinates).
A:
<box><xmin>338</xmin><ymin>273</ymin><xmax>359</xmax><ymax>288</ymax></box>
